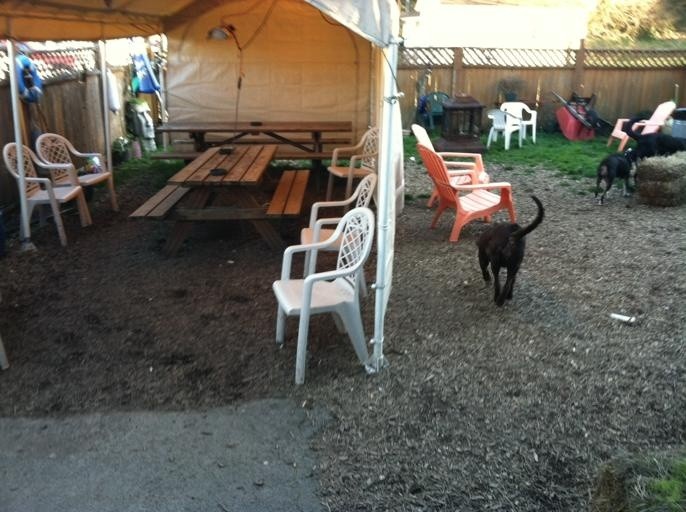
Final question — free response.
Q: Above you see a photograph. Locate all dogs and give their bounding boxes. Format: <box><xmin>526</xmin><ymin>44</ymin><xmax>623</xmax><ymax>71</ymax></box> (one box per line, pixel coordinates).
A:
<box><xmin>474</xmin><ymin>194</ymin><xmax>545</xmax><ymax>307</ymax></box>
<box><xmin>595</xmin><ymin>150</ymin><xmax>641</xmax><ymax>206</ymax></box>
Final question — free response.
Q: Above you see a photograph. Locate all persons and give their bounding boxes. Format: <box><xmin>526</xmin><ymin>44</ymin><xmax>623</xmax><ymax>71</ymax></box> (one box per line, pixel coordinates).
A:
<box><xmin>130</xmin><ymin>99</ymin><xmax>157</xmax><ymax>151</ymax></box>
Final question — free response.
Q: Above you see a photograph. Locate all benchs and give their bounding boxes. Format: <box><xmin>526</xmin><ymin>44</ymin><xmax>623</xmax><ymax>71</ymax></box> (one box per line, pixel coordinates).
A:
<box><xmin>172</xmin><ymin>137</ymin><xmax>351</xmax><ymax>144</ymax></box>
<box><xmin>129</xmin><ymin>185</ymin><xmax>190</xmax><ymax>217</ymax></box>
<box><xmin>267</xmin><ymin>170</ymin><xmax>310</xmax><ymax>214</ymax></box>
<box><xmin>151</xmin><ymin>151</ymin><xmax>351</xmax><ymax>159</ymax></box>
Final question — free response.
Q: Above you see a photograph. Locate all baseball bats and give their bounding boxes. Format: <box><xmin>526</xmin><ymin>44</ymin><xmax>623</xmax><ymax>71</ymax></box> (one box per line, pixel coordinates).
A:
<box><xmin>552</xmin><ymin>92</ymin><xmax>592</xmax><ymax>130</ymax></box>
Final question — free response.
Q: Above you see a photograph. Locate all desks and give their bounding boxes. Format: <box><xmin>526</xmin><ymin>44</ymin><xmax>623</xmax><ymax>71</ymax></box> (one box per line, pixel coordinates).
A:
<box><xmin>168</xmin><ymin>144</ymin><xmax>278</xmax><ymax>186</ymax></box>
<box><xmin>442</xmin><ymin>102</ymin><xmax>486</xmax><ymax>140</ymax></box>
<box><xmin>159</xmin><ymin>121</ymin><xmax>352</xmax><ymax>132</ymax></box>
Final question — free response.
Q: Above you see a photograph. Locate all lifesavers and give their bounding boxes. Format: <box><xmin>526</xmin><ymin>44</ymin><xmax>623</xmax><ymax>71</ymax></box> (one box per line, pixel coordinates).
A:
<box><xmin>14</xmin><ymin>54</ymin><xmax>43</xmax><ymax>102</ymax></box>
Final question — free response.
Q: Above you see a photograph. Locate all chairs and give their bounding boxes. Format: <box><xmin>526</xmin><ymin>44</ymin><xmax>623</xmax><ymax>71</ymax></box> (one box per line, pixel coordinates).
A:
<box><xmin>416</xmin><ymin>143</ymin><xmax>516</xmax><ymax>242</ymax></box>
<box><xmin>423</xmin><ymin>92</ymin><xmax>450</xmax><ymax>129</ymax></box>
<box><xmin>411</xmin><ymin>124</ymin><xmax>488</xmax><ymax>209</ymax></box>
<box><xmin>487</xmin><ymin>109</ymin><xmax>522</xmax><ymax>150</ymax></box>
<box><xmin>36</xmin><ymin>133</ymin><xmax>119</xmax><ymax>225</ymax></box>
<box><xmin>3</xmin><ymin>142</ymin><xmax>87</xmax><ymax>247</ymax></box>
<box><xmin>501</xmin><ymin>102</ymin><xmax>537</xmax><ymax>143</ymax></box>
<box><xmin>300</xmin><ymin>173</ymin><xmax>376</xmax><ymax>280</ymax></box>
<box><xmin>607</xmin><ymin>101</ymin><xmax>675</xmax><ymax>153</ymax></box>
<box><xmin>326</xmin><ymin>128</ymin><xmax>379</xmax><ymax>199</ymax></box>
<box><xmin>272</xmin><ymin>207</ymin><xmax>375</xmax><ymax>383</ymax></box>
